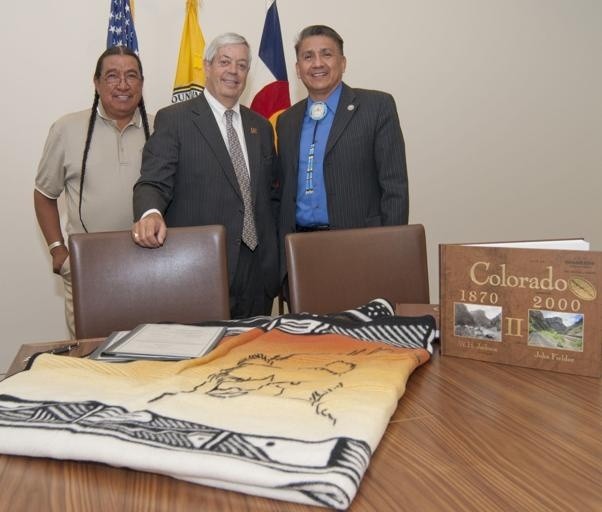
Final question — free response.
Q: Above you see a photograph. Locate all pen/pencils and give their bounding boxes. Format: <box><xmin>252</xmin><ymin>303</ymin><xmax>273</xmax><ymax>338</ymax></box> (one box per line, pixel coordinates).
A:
<box><xmin>23</xmin><ymin>343</ymin><xmax>80</xmax><ymax>362</ymax></box>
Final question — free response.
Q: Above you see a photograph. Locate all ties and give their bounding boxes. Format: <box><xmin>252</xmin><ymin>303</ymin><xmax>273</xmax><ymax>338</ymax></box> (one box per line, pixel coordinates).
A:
<box><xmin>224</xmin><ymin>110</ymin><xmax>258</xmax><ymax>252</ymax></box>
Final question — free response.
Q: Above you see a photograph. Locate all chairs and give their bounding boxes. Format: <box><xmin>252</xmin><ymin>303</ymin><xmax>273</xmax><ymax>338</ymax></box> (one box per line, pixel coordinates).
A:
<box><xmin>284</xmin><ymin>222</ymin><xmax>429</xmax><ymax>315</ymax></box>
<box><xmin>68</xmin><ymin>224</ymin><xmax>231</xmax><ymax>339</ymax></box>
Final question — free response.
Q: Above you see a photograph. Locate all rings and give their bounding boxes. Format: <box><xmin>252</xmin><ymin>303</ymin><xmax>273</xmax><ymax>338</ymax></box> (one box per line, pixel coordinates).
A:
<box><xmin>133</xmin><ymin>233</ymin><xmax>140</xmax><ymax>237</ymax></box>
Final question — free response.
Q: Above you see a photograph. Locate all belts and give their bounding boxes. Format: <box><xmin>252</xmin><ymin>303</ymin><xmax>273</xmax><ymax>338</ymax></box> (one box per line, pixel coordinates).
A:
<box><xmin>293</xmin><ymin>223</ymin><xmax>328</xmax><ymax>232</ymax></box>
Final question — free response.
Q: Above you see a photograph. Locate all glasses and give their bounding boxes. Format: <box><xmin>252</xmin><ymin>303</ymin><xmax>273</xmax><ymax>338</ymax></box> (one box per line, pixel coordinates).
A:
<box><xmin>99</xmin><ymin>75</ymin><xmax>142</xmax><ymax>86</ymax></box>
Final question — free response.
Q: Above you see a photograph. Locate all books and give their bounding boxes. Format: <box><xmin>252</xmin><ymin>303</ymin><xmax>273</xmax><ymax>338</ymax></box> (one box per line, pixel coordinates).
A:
<box><xmin>437</xmin><ymin>237</ymin><xmax>602</xmax><ymax>380</ymax></box>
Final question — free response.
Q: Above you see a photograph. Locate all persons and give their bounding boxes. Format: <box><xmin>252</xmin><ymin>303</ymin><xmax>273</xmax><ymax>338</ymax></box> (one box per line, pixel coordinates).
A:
<box><xmin>31</xmin><ymin>45</ymin><xmax>156</xmax><ymax>340</ymax></box>
<box><xmin>131</xmin><ymin>30</ymin><xmax>282</xmax><ymax>318</ymax></box>
<box><xmin>275</xmin><ymin>24</ymin><xmax>409</xmax><ymax>315</ymax></box>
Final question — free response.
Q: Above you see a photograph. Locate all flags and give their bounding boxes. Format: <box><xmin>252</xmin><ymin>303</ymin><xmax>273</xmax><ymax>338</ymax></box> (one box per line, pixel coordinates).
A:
<box><xmin>106</xmin><ymin>0</ymin><xmax>138</xmax><ymax>57</ymax></box>
<box><xmin>170</xmin><ymin>0</ymin><xmax>208</xmax><ymax>105</ymax></box>
<box><xmin>240</xmin><ymin>0</ymin><xmax>291</xmax><ymax>192</ymax></box>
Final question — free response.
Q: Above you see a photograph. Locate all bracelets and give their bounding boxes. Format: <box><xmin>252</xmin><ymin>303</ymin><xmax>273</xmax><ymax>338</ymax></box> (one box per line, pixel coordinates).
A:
<box><xmin>48</xmin><ymin>241</ymin><xmax>65</xmax><ymax>253</ymax></box>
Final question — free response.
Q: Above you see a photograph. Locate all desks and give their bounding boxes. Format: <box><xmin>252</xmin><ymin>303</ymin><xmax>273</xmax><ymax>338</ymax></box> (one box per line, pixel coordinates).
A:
<box><xmin>0</xmin><ymin>329</ymin><xmax>602</xmax><ymax>512</ymax></box>
